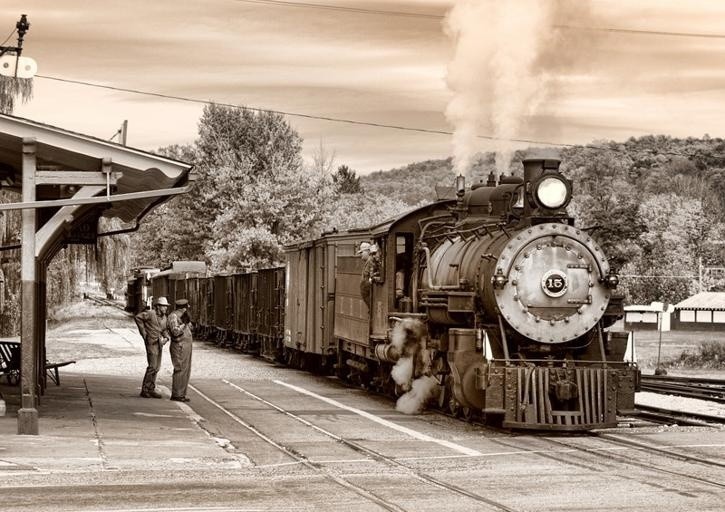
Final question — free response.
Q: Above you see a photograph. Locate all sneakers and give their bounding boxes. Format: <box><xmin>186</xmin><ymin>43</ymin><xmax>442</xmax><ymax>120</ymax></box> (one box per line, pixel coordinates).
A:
<box><xmin>170</xmin><ymin>396</ymin><xmax>190</xmax><ymax>402</ymax></box>
<box><xmin>140</xmin><ymin>391</ymin><xmax>162</xmax><ymax>398</ymax></box>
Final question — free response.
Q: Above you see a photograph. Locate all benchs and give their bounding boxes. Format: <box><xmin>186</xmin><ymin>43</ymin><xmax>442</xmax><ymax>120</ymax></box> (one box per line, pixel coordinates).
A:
<box><xmin>0</xmin><ymin>341</ymin><xmax>75</xmax><ymax>386</ymax></box>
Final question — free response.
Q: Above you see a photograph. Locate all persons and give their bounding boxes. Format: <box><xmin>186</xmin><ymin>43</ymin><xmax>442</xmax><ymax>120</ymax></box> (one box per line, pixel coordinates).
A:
<box><xmin>369</xmin><ymin>244</ymin><xmax>382</xmax><ymax>281</ymax></box>
<box><xmin>134</xmin><ymin>296</ymin><xmax>171</xmax><ymax>399</ymax></box>
<box><xmin>356</xmin><ymin>241</ymin><xmax>371</xmax><ymax>311</ymax></box>
<box><xmin>166</xmin><ymin>299</ymin><xmax>193</xmax><ymax>402</ymax></box>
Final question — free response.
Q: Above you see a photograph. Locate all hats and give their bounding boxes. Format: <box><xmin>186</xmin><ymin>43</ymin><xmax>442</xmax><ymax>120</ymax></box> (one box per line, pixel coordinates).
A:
<box><xmin>175</xmin><ymin>299</ymin><xmax>191</xmax><ymax>308</ymax></box>
<box><xmin>154</xmin><ymin>297</ymin><xmax>171</xmax><ymax>307</ymax></box>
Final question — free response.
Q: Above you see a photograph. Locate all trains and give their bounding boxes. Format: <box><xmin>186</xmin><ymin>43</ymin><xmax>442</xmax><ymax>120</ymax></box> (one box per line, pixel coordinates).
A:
<box><xmin>123</xmin><ymin>158</ymin><xmax>642</xmax><ymax>432</ymax></box>
